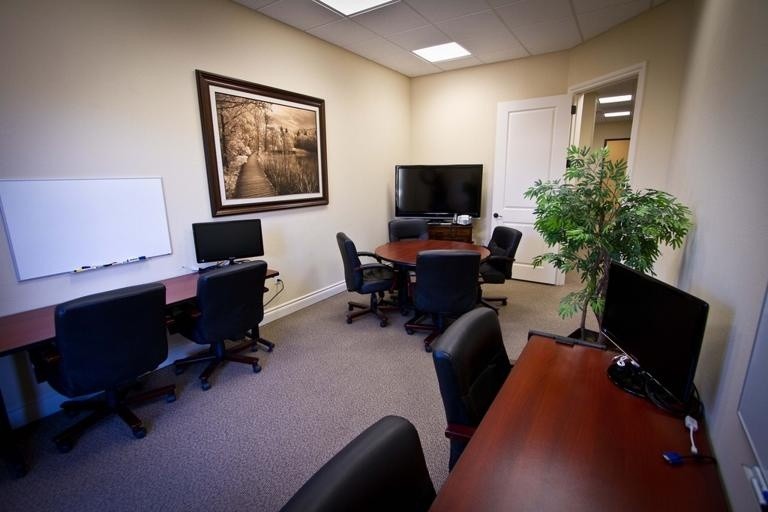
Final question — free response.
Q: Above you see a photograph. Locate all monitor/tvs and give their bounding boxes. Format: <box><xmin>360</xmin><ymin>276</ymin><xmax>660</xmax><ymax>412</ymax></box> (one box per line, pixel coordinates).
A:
<box><xmin>600</xmin><ymin>261</ymin><xmax>709</xmax><ymax>404</ymax></box>
<box><xmin>192</xmin><ymin>219</ymin><xmax>264</xmax><ymax>263</ymax></box>
<box><xmin>395</xmin><ymin>164</ymin><xmax>483</xmax><ymax>226</ymax></box>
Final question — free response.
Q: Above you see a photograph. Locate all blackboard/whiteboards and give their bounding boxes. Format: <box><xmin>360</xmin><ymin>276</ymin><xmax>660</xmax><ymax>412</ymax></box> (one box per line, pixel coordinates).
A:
<box><xmin>736</xmin><ymin>283</ymin><xmax>768</xmax><ymax>487</ymax></box>
<box><xmin>0</xmin><ymin>177</ymin><xmax>173</xmax><ymax>283</ymax></box>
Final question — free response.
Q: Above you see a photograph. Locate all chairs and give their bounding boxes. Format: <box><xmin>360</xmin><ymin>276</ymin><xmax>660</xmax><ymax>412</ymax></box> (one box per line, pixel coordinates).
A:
<box><xmin>281</xmin><ymin>414</ymin><xmax>440</xmax><ymax>511</ymax></box>
<box><xmin>430</xmin><ymin>308</ymin><xmax>518</xmax><ymax>473</ymax></box>
<box><xmin>336</xmin><ymin>218</ymin><xmax>523</xmax><ymax>352</ymax></box>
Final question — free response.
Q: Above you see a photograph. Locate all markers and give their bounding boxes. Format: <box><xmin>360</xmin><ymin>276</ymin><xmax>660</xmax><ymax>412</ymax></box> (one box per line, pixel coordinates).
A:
<box><xmin>751</xmin><ymin>478</ymin><xmax>767</xmax><ymax>512</ymax></box>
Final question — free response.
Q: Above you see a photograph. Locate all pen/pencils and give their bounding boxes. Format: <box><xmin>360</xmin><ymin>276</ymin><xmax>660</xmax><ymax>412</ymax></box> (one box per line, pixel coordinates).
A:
<box><xmin>74</xmin><ymin>256</ymin><xmax>146</xmax><ymax>273</ymax></box>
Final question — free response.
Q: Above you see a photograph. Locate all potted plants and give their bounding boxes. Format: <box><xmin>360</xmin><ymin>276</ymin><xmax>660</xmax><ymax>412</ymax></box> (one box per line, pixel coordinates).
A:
<box><xmin>526</xmin><ymin>145</ymin><xmax>691</xmax><ymax>349</ymax></box>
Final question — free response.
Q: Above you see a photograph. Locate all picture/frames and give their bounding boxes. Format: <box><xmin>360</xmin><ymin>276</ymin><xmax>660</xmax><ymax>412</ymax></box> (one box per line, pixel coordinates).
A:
<box><xmin>196</xmin><ymin>69</ymin><xmax>329</xmax><ymax>218</ymax></box>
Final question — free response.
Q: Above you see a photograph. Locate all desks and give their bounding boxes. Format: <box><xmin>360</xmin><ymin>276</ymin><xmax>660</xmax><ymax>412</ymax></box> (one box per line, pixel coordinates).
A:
<box><xmin>432</xmin><ymin>336</ymin><xmax>731</xmax><ymax>512</ymax></box>
<box><xmin>0</xmin><ymin>261</ymin><xmax>279</xmax><ymax>479</ymax></box>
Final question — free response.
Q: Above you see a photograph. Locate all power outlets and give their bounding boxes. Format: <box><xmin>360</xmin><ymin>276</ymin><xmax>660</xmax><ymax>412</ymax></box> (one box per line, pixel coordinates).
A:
<box><xmin>274</xmin><ymin>275</ymin><xmax>281</xmax><ymax>284</ymax></box>
